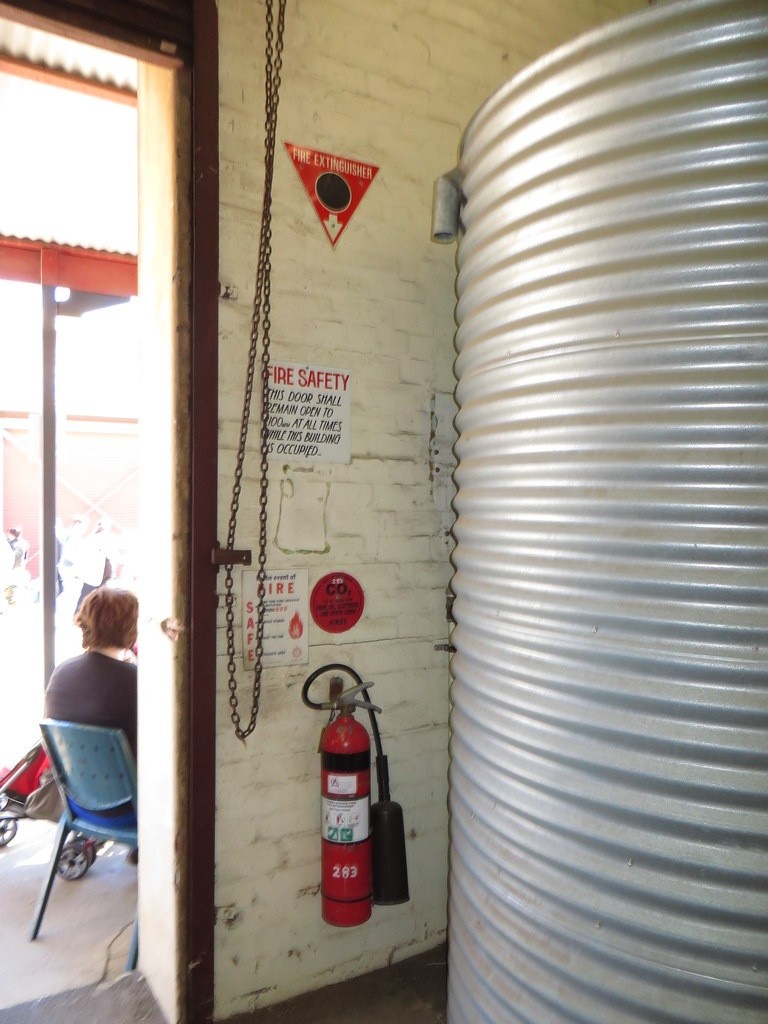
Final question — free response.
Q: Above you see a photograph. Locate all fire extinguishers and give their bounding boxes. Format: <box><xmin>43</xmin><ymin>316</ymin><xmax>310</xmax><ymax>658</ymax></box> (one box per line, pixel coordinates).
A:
<box><xmin>299</xmin><ymin>661</ymin><xmax>412</xmax><ymax>929</ymax></box>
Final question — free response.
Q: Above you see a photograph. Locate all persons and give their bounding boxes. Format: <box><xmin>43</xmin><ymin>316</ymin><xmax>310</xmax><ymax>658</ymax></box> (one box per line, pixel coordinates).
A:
<box><xmin>0</xmin><ymin>522</ymin><xmax>113</xmax><ymax>616</ymax></box>
<box><xmin>45</xmin><ymin>587</ymin><xmax>138</xmax><ymax>818</ymax></box>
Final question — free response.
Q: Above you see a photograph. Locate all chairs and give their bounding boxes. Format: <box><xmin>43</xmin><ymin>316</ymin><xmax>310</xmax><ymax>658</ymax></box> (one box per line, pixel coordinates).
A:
<box><xmin>27</xmin><ymin>717</ymin><xmax>139</xmax><ymax>971</ymax></box>
